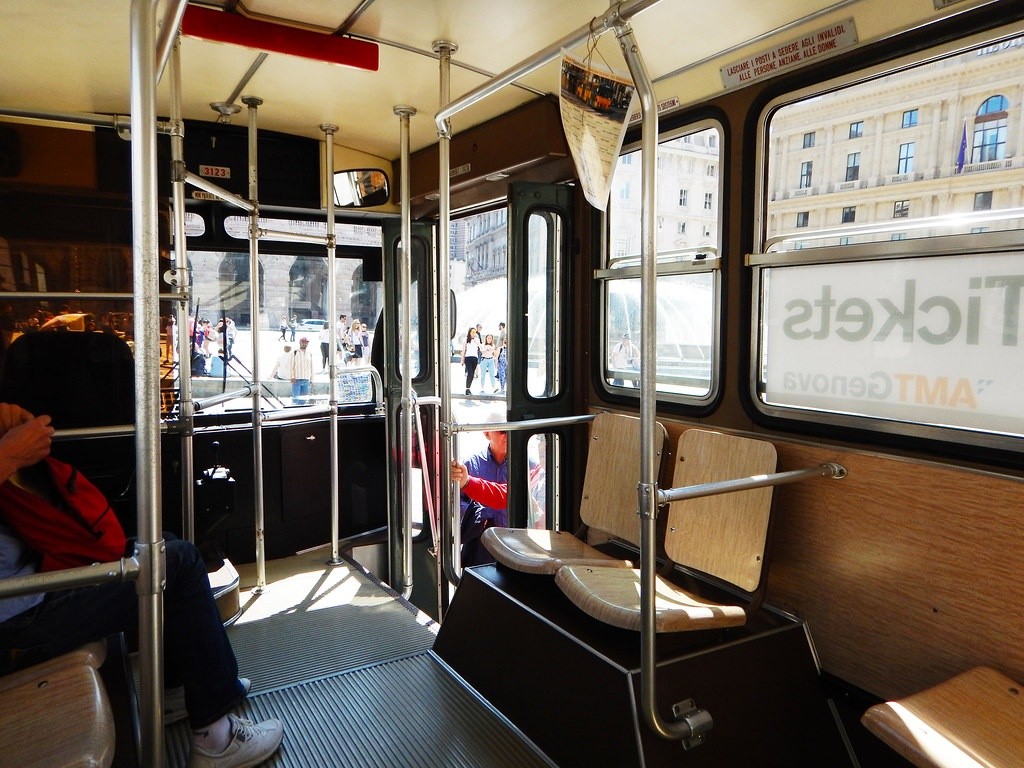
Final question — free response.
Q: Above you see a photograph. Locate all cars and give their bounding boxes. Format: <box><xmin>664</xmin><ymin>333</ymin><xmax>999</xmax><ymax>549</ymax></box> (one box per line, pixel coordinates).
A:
<box><xmin>297</xmin><ymin>318</ymin><xmax>325</xmax><ymax>329</ymax></box>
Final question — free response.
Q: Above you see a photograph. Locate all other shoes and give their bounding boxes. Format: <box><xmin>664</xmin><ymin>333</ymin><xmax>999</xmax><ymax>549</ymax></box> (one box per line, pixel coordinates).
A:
<box><xmin>285</xmin><ymin>340</ymin><xmax>287</xmax><ymax>342</ymax></box>
<box><xmin>465</xmin><ymin>389</ymin><xmax>471</xmax><ymax>396</ymax></box>
<box><xmin>494</xmin><ymin>389</ymin><xmax>498</xmax><ymax>393</ymax></box>
<box><xmin>481</xmin><ymin>391</ymin><xmax>484</xmax><ymax>394</ymax></box>
<box><xmin>290</xmin><ymin>340</ymin><xmax>293</xmax><ymax>342</ymax></box>
<box><xmin>294</xmin><ymin>340</ymin><xmax>295</xmax><ymax>341</ymax></box>
<box><xmin>278</xmin><ymin>339</ymin><xmax>281</xmax><ymax>342</ymax></box>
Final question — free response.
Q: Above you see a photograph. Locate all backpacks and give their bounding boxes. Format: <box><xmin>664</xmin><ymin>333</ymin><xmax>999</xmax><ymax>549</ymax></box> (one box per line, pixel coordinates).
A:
<box><xmin>0</xmin><ymin>457</ymin><xmax>127</xmax><ymax>573</ymax></box>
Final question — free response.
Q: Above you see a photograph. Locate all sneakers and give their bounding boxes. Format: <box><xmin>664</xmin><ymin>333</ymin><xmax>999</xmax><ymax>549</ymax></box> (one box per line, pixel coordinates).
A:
<box><xmin>164</xmin><ymin>678</ymin><xmax>250</xmax><ymax>724</ymax></box>
<box><xmin>187</xmin><ymin>713</ymin><xmax>283</xmax><ymax>768</ymax></box>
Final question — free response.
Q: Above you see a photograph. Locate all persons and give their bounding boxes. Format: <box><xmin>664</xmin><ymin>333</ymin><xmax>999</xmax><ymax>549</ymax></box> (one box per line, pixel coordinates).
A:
<box><xmin>460</xmin><ymin>412</ymin><xmax>537</xmax><ymax>568</ymax></box>
<box><xmin>0</xmin><ymin>402</ymin><xmax>283</xmax><ymax>768</ymax></box>
<box><xmin>451</xmin><ymin>433</ymin><xmax>545</xmax><ymax>529</ymax></box>
<box><xmin>268</xmin><ymin>345</ymin><xmax>293</xmax><ymax>380</ymax></box>
<box><xmin>278</xmin><ymin>316</ymin><xmax>288</xmax><ymax>342</ymax></box>
<box><xmin>320</xmin><ymin>314</ymin><xmax>370</xmax><ymax>375</ymax></box>
<box><xmin>14</xmin><ymin>305</ymin><xmax>173</xmax><ymax>365</ymax></box>
<box><xmin>461</xmin><ymin>322</ymin><xmax>507</xmax><ymax>396</ymax></box>
<box><xmin>289</xmin><ymin>336</ymin><xmax>313</xmax><ymax>405</ymax></box>
<box><xmin>189</xmin><ymin>315</ymin><xmax>238</xmax><ymax>359</ymax></box>
<box><xmin>610</xmin><ymin>333</ymin><xmax>640</xmax><ymax>388</ymax></box>
<box><xmin>289</xmin><ymin>315</ymin><xmax>297</xmax><ymax>342</ymax></box>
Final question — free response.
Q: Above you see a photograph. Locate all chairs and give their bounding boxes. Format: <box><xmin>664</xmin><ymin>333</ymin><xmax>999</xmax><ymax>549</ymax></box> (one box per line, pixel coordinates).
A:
<box><xmin>861</xmin><ymin>666</ymin><xmax>1024</xmax><ymax>768</ymax></box>
<box><xmin>0</xmin><ymin>330</ymin><xmax>173</xmax><ymax>505</ymax></box>
<box><xmin>482</xmin><ymin>412</ymin><xmax>670</xmax><ymax>573</ymax></box>
<box><xmin>556</xmin><ymin>428</ymin><xmax>777</xmax><ymax>633</ymax></box>
<box><xmin>0</xmin><ymin>638</ymin><xmax>116</xmax><ymax>768</ymax></box>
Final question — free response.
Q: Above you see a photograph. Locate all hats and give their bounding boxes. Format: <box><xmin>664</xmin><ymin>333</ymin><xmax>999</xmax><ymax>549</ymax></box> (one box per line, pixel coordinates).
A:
<box><xmin>300</xmin><ymin>337</ymin><xmax>308</xmax><ymax>343</ymax></box>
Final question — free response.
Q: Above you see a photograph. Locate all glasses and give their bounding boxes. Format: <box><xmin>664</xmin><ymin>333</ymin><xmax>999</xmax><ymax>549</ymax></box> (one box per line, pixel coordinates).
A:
<box><xmin>498</xmin><ymin>431</ymin><xmax>506</xmax><ymax>434</ymax></box>
